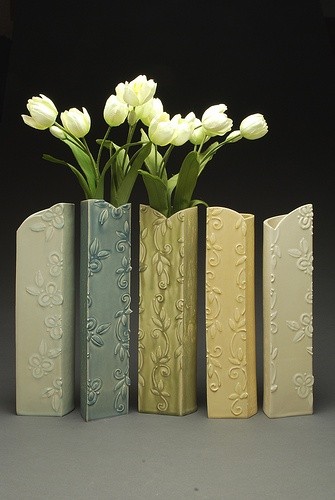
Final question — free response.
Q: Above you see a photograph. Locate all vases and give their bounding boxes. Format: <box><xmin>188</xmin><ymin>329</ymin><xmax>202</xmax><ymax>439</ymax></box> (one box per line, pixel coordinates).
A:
<box><xmin>204</xmin><ymin>206</ymin><xmax>257</xmax><ymax>419</ymax></box>
<box><xmin>262</xmin><ymin>204</ymin><xmax>315</xmax><ymax>418</ymax></box>
<box><xmin>137</xmin><ymin>203</ymin><xmax>199</xmax><ymax>417</ymax></box>
<box><xmin>14</xmin><ymin>203</ymin><xmax>75</xmax><ymax>417</ymax></box>
<box><xmin>80</xmin><ymin>198</ymin><xmax>131</xmax><ymax>421</ymax></box>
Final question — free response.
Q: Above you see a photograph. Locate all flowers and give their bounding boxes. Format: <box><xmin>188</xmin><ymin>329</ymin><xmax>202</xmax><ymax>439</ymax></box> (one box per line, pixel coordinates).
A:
<box><xmin>139</xmin><ymin>103</ymin><xmax>268</xmax><ymax>217</ymax></box>
<box><xmin>21</xmin><ymin>73</ymin><xmax>194</xmax><ymax>209</ymax></box>
